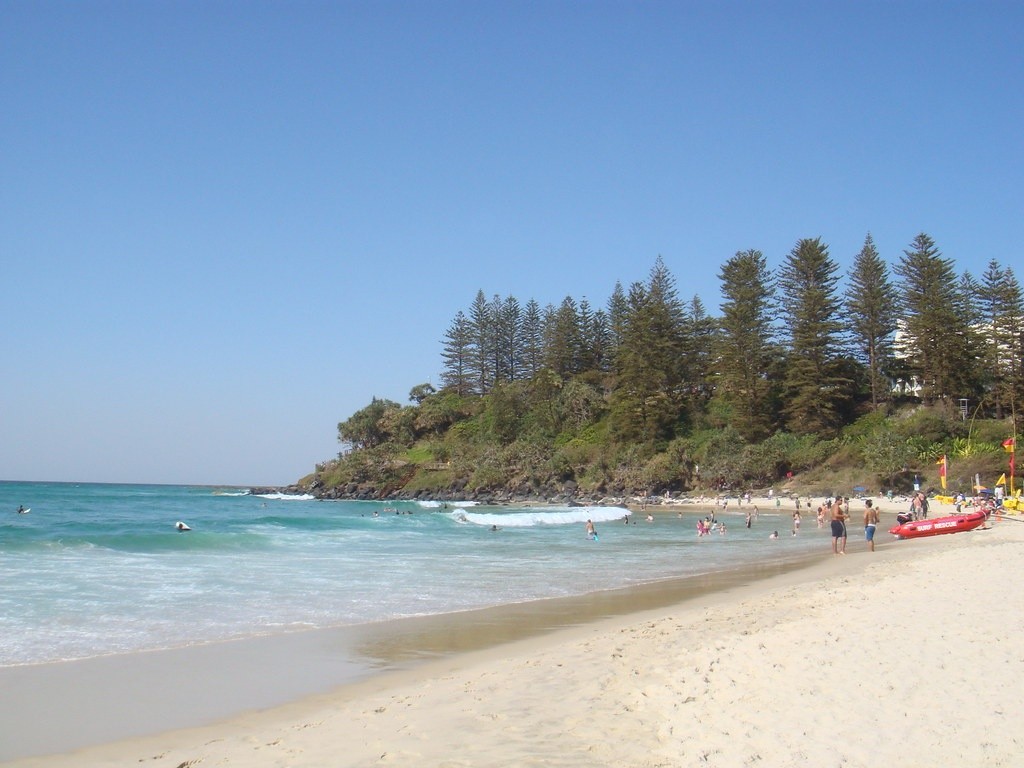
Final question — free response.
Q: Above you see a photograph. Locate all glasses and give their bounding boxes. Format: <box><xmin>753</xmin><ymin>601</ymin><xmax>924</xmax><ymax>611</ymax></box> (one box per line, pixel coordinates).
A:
<box><xmin>839</xmin><ymin>500</ymin><xmax>842</xmax><ymax>501</ymax></box>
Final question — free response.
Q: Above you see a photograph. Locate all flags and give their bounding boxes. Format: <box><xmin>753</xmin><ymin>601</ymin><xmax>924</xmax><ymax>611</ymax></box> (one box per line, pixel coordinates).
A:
<box><xmin>936</xmin><ymin>456</ymin><xmax>947</xmax><ymax>492</ymax></box>
<box><xmin>1002</xmin><ymin>438</ymin><xmax>1014</xmax><ymax>453</ymax></box>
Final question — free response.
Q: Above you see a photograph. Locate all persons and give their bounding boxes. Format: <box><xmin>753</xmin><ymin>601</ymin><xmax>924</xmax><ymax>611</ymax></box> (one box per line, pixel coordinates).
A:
<box><xmin>955</xmin><ymin>490</ymin><xmax>964</xmax><ymax>513</ymax></box>
<box><xmin>875</xmin><ymin>506</ymin><xmax>880</xmax><ymax>523</ymax></box>
<box><xmin>17</xmin><ymin>505</ymin><xmax>24</xmax><ymax>513</ymax></box>
<box><xmin>864</xmin><ymin>500</ymin><xmax>880</xmax><ymax>552</ymax></box>
<box><xmin>585</xmin><ymin>519</ymin><xmax>601</xmax><ymax>541</ymax></box>
<box><xmin>696</xmin><ymin>510</ymin><xmax>727</xmax><ymax>537</ymax></box>
<box><xmin>639</xmin><ymin>489</ymin><xmax>883</xmax><ymax>528</ymax></box>
<box><xmin>897</xmin><ymin>492</ymin><xmax>929</xmax><ymax>527</ymax></box>
<box><xmin>995</xmin><ymin>486</ymin><xmax>1004</xmax><ymax>508</ymax></box>
<box><xmin>623</xmin><ymin>514</ymin><xmax>629</xmax><ymax>526</ymax></box>
<box><xmin>372</xmin><ymin>506</ymin><xmax>413</xmax><ymax>519</ymax></box>
<box><xmin>177</xmin><ymin>523</ymin><xmax>186</xmax><ymax>531</ymax></box>
<box><xmin>770</xmin><ymin>530</ymin><xmax>796</xmax><ymax>540</ymax></box>
<box><xmin>488</xmin><ymin>525</ymin><xmax>499</xmax><ymax>532</ymax></box>
<box><xmin>830</xmin><ymin>495</ymin><xmax>848</xmax><ymax>556</ymax></box>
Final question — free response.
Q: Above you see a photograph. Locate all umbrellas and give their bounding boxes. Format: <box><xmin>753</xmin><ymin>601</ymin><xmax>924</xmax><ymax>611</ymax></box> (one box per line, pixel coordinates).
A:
<box><xmin>973</xmin><ymin>485</ymin><xmax>995</xmax><ymax>496</ymax></box>
<box><xmin>853</xmin><ymin>487</ymin><xmax>865</xmax><ymax>491</ymax></box>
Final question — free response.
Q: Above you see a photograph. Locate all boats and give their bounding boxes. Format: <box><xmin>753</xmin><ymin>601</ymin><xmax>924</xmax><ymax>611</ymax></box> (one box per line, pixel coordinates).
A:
<box><xmin>890</xmin><ymin>510</ymin><xmax>990</xmax><ymax>540</ymax></box>
